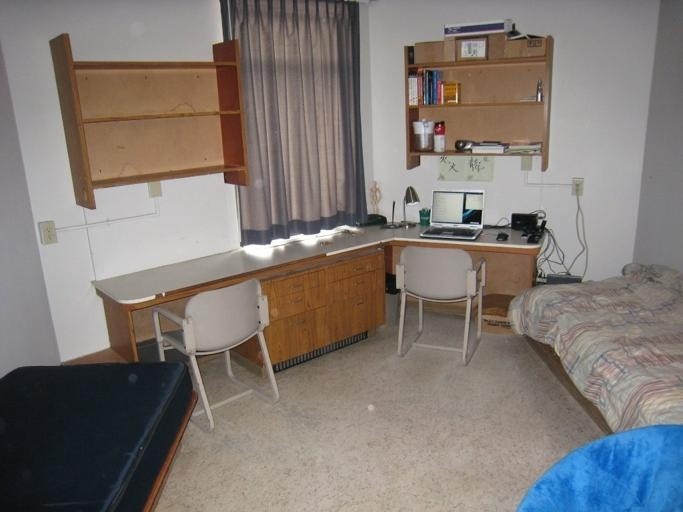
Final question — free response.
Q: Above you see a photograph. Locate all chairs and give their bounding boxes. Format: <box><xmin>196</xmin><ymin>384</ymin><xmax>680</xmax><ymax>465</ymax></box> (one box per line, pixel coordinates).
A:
<box><xmin>152</xmin><ymin>278</ymin><xmax>279</xmax><ymax>434</ymax></box>
<box><xmin>394</xmin><ymin>246</ymin><xmax>486</xmax><ymax>366</ymax></box>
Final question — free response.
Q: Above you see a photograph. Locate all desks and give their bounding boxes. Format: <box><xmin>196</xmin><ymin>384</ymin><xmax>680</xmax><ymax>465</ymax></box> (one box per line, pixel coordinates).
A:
<box><xmin>517</xmin><ymin>424</ymin><xmax>683</xmax><ymax>512</ymax></box>
<box><xmin>1</xmin><ymin>361</ymin><xmax>198</xmax><ymax>512</ymax></box>
<box><xmin>91</xmin><ymin>221</ymin><xmax>393</xmax><ymax>380</ymax></box>
<box><xmin>389</xmin><ymin>223</ymin><xmax>546</xmax><ymax>319</ymax></box>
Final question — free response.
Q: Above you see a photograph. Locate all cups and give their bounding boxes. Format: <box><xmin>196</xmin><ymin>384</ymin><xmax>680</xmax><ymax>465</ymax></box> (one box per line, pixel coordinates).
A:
<box><xmin>418</xmin><ymin>208</ymin><xmax>429</xmax><ymax>224</ymax></box>
<box><xmin>411</xmin><ymin>118</ymin><xmax>433</xmax><ymax>152</ymax></box>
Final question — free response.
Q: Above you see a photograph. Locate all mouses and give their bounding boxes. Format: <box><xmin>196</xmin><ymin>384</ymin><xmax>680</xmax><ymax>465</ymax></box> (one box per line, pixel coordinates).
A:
<box><xmin>497</xmin><ymin>233</ymin><xmax>507</xmax><ymax>240</ymax></box>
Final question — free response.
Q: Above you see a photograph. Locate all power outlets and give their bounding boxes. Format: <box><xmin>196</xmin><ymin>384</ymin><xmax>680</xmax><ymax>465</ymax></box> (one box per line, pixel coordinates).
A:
<box><xmin>38</xmin><ymin>220</ymin><xmax>58</xmax><ymax>245</ymax></box>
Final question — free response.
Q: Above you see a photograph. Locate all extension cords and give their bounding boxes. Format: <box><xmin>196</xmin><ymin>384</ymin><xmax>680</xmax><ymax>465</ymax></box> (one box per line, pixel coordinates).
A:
<box><xmin>536</xmin><ymin>277</ymin><xmax>547</xmax><ymax>283</ymax></box>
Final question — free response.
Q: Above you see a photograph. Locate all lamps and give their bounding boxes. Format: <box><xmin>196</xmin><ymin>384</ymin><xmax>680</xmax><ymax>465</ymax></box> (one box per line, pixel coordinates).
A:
<box><xmin>397</xmin><ymin>186</ymin><xmax>421</xmax><ymax>230</ymax></box>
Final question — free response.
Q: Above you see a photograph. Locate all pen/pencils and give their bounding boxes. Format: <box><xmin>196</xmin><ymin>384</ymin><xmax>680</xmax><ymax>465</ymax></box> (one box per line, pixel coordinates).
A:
<box><xmin>420</xmin><ymin>208</ymin><xmax>430</xmax><ymax>216</ymax></box>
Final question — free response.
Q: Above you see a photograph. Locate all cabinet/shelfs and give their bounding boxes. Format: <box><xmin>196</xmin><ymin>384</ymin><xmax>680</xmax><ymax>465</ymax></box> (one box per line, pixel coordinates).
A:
<box><xmin>48</xmin><ymin>32</ymin><xmax>250</xmax><ymax>210</ymax></box>
<box><xmin>404</xmin><ymin>33</ymin><xmax>554</xmax><ymax>171</ymax></box>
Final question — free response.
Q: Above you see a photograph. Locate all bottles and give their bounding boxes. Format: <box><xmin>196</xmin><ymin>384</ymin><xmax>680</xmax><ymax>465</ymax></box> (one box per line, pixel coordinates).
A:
<box><xmin>535</xmin><ymin>80</ymin><xmax>542</xmax><ymax>101</ymax></box>
<box><xmin>434</xmin><ymin>122</ymin><xmax>445</xmax><ymax>152</ymax></box>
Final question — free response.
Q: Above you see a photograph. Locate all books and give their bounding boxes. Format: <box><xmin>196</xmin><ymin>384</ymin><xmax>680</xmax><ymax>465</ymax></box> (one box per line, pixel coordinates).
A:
<box><xmin>408</xmin><ymin>69</ymin><xmax>460</xmax><ymax>105</ymax></box>
<box><xmin>471</xmin><ymin>141</ymin><xmax>542</xmax><ymax>154</ymax></box>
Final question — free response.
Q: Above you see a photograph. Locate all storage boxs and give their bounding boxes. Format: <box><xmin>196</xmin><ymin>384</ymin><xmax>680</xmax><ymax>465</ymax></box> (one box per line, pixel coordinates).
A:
<box><xmin>504</xmin><ymin>37</ymin><xmax>547</xmax><ymax>59</ymax></box>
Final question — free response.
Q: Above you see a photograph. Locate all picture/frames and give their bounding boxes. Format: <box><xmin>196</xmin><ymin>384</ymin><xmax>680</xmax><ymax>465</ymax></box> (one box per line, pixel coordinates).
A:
<box><xmin>456</xmin><ymin>36</ymin><xmax>488</xmax><ymax>61</ymax></box>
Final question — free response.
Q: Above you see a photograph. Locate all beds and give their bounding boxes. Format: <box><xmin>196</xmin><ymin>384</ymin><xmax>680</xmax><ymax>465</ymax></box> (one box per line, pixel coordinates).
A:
<box><xmin>506</xmin><ymin>264</ymin><xmax>683</xmax><ymax>434</ymax></box>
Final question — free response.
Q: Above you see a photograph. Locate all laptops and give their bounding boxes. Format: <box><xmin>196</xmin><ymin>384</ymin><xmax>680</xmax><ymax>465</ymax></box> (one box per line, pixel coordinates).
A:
<box><xmin>420</xmin><ymin>190</ymin><xmax>485</xmax><ymax>241</ymax></box>
